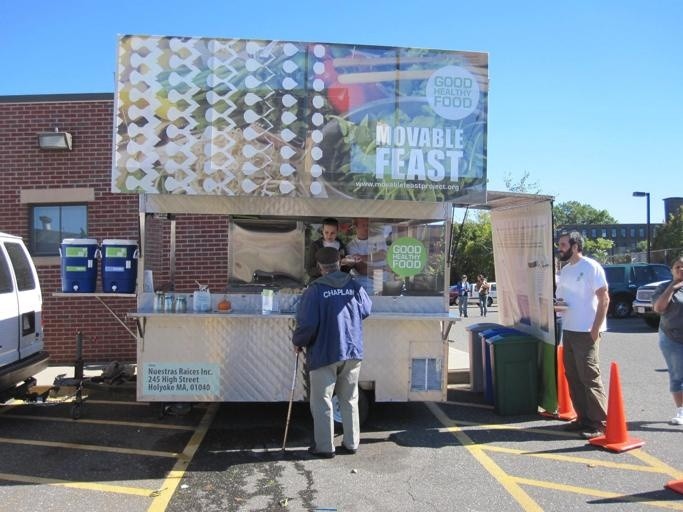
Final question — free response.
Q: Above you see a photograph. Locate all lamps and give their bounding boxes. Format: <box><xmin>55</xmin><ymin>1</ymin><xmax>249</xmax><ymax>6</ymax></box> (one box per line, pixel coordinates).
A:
<box><xmin>35</xmin><ymin>126</ymin><xmax>74</xmax><ymax>155</ymax></box>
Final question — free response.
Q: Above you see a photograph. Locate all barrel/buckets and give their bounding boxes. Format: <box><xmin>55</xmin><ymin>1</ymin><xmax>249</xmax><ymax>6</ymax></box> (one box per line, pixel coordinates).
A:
<box><xmin>59</xmin><ymin>239</ymin><xmax>98</xmax><ymax>293</ymax></box>
<box><xmin>99</xmin><ymin>238</ymin><xmax>139</xmax><ymax>293</ymax></box>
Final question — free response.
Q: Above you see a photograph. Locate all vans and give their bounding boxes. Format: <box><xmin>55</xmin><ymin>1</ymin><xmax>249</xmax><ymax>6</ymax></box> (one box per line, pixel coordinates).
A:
<box><xmin>0</xmin><ymin>228</ymin><xmax>58</xmax><ymax>408</ymax></box>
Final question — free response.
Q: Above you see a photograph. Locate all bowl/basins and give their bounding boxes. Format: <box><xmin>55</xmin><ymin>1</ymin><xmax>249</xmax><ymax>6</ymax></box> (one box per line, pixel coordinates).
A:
<box><xmin>371</xmin><ymin>51</ymin><xmax>405</xmax><ymax>98</ymax></box>
<box><xmin>310</xmin><ymin>96</ymin><xmax>486</xmax><ymax>203</ymax></box>
<box><xmin>383</xmin><ymin>279</ymin><xmax>403</xmax><ymax>295</ymax></box>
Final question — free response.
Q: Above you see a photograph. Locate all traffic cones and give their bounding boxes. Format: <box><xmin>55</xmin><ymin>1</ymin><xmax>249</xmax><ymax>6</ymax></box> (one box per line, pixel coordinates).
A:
<box><xmin>587</xmin><ymin>360</ymin><xmax>645</xmax><ymax>455</ymax></box>
<box><xmin>663</xmin><ymin>478</ymin><xmax>683</xmax><ymax>494</ymax></box>
<box><xmin>540</xmin><ymin>339</ymin><xmax>577</xmax><ymax>420</ymax></box>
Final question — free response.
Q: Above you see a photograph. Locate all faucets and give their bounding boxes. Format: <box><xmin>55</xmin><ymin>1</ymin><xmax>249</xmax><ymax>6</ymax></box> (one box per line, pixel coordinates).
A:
<box><xmin>398</xmin><ymin>278</ymin><xmax>407</xmax><ymax>297</ymax></box>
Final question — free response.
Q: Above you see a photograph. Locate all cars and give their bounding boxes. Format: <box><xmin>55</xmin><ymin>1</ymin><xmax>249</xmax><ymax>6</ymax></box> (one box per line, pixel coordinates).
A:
<box><xmin>449</xmin><ymin>284</ymin><xmax>460</xmax><ymax>307</ymax></box>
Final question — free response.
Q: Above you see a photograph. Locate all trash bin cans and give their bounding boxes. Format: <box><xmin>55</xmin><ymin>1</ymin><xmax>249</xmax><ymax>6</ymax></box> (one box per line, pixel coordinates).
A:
<box><xmin>465</xmin><ymin>323</ymin><xmax>540</xmax><ymax>416</ymax></box>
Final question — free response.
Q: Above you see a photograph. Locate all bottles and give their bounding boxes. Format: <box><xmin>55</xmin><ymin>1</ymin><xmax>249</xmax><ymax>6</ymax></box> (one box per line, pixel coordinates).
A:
<box><xmin>143</xmin><ymin>270</ymin><xmax>153</xmax><ymax>293</ymax></box>
<box><xmin>432</xmin><ymin>272</ymin><xmax>439</xmax><ymax>291</ymax></box>
<box><xmin>155</xmin><ymin>291</ymin><xmax>187</xmax><ymax>313</ymax></box>
<box><xmin>404</xmin><ymin>275</ymin><xmax>411</xmax><ymax>290</ymax></box>
<box><xmin>261</xmin><ymin>289</ymin><xmax>274</xmax><ymax>315</ymax></box>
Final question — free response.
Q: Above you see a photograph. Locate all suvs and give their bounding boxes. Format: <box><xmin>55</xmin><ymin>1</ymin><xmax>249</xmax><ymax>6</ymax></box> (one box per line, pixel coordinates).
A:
<box><xmin>601</xmin><ymin>261</ymin><xmax>672</xmax><ymax>319</ymax></box>
<box><xmin>630</xmin><ymin>279</ymin><xmax>672</xmax><ymax>328</ymax></box>
<box><xmin>460</xmin><ymin>280</ymin><xmax>497</xmax><ymax>307</ymax></box>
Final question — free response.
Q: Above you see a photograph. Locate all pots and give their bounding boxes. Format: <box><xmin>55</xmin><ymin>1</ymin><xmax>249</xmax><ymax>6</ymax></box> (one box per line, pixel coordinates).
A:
<box><xmin>412</xmin><ymin>272</ymin><xmax>434</xmax><ymax>290</ymax></box>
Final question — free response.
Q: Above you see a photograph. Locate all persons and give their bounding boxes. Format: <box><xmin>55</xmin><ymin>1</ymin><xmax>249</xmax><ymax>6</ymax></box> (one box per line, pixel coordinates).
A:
<box><xmin>344</xmin><ymin>217</ymin><xmax>386</xmax><ymax>295</ymax></box>
<box><xmin>651</xmin><ymin>257</ymin><xmax>683</xmax><ymax>424</ymax></box>
<box><xmin>553</xmin><ymin>231</ymin><xmax>610</xmax><ymax>438</ymax></box>
<box><xmin>475</xmin><ymin>274</ymin><xmax>489</xmax><ymax>316</ymax></box>
<box><xmin>292</xmin><ymin>247</ymin><xmax>372</xmax><ymax>457</ymax></box>
<box><xmin>457</xmin><ymin>275</ymin><xmax>469</xmax><ymax>317</ymax></box>
<box><xmin>304</xmin><ymin>218</ymin><xmax>361</xmax><ymax>284</ymax></box>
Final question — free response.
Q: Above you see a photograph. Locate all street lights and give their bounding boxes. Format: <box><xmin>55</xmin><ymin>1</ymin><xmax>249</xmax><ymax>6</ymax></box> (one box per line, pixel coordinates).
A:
<box><xmin>632</xmin><ymin>191</ymin><xmax>652</xmax><ymax>262</ymax></box>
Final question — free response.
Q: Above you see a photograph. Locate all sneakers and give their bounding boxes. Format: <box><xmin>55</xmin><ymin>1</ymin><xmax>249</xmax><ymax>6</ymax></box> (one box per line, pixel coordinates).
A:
<box><xmin>558</xmin><ymin>420</ymin><xmax>584</xmax><ymax>432</ymax></box>
<box><xmin>582</xmin><ymin>428</ymin><xmax>604</xmax><ymax>438</ymax></box>
<box><xmin>671</xmin><ymin>408</ymin><xmax>682</xmax><ymax>424</ymax></box>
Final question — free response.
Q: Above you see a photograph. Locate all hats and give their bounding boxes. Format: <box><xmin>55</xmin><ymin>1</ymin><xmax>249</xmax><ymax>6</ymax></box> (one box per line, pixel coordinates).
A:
<box><xmin>314</xmin><ymin>247</ymin><xmax>339</xmax><ymax>264</ymax></box>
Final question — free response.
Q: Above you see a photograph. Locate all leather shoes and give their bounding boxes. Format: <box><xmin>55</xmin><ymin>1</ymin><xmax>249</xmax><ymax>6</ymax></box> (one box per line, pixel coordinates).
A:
<box><xmin>307</xmin><ymin>447</ymin><xmax>335</xmax><ymax>458</ymax></box>
<box><xmin>341</xmin><ymin>442</ymin><xmax>355</xmax><ymax>454</ymax></box>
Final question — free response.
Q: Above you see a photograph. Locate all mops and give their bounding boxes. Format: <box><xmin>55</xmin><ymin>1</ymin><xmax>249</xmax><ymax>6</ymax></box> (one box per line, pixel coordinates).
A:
<box><xmin>248</xmin><ymin>351</ymin><xmax>303</xmax><ymax>461</ymax></box>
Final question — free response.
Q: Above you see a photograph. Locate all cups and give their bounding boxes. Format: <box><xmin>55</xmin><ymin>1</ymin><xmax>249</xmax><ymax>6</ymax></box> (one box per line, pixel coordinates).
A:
<box><xmin>193</xmin><ymin>288</ymin><xmax>213</xmax><ymax>313</ymax></box>
<box><xmin>373</xmin><ymin>269</ymin><xmax>384</xmax><ymax>296</ymax></box>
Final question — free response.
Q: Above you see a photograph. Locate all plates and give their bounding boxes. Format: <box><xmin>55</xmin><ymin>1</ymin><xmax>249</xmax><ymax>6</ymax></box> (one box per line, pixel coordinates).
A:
<box><xmin>217</xmin><ymin>309</ymin><xmax>233</xmax><ymax>313</ymax></box>
